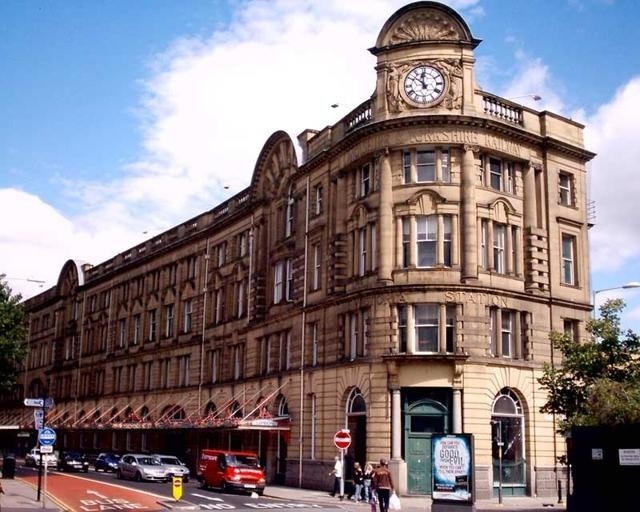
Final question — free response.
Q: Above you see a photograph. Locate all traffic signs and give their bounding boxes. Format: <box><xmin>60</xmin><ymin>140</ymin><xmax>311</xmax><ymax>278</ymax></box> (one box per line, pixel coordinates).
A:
<box><xmin>24</xmin><ymin>399</ymin><xmax>43</xmax><ymax>406</ymax></box>
<box><xmin>45</xmin><ymin>398</ymin><xmax>53</xmax><ymax>405</ymax></box>
<box><xmin>35</xmin><ymin>410</ymin><xmax>43</xmax><ymax>430</ymax></box>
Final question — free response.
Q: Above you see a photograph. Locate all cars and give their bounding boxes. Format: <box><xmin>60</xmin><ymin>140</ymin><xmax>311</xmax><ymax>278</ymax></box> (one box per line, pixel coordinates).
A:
<box><xmin>25</xmin><ymin>447</ymin><xmax>191</xmax><ymax>485</ymax></box>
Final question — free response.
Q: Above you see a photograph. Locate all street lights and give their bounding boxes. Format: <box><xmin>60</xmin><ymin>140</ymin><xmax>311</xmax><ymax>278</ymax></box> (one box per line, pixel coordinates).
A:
<box><xmin>593</xmin><ymin>282</ymin><xmax>640</xmax><ymax>342</ymax></box>
<box><xmin>489</xmin><ymin>419</ymin><xmax>503</xmax><ymax>503</ymax></box>
<box><xmin>1</xmin><ymin>276</ymin><xmax>45</xmax><ymax>286</ymax></box>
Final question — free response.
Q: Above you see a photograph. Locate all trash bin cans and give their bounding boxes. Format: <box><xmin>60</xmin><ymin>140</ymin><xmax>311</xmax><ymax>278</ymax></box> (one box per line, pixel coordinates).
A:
<box><xmin>2</xmin><ymin>455</ymin><xmax>16</xmax><ymax>479</ymax></box>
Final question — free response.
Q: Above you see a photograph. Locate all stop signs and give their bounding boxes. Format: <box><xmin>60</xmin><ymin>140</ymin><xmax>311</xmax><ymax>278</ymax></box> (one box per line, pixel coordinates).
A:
<box><xmin>334</xmin><ymin>430</ymin><xmax>352</xmax><ymax>448</ymax></box>
<box><xmin>174</xmin><ymin>479</ymin><xmax>180</xmax><ymax>487</ymax></box>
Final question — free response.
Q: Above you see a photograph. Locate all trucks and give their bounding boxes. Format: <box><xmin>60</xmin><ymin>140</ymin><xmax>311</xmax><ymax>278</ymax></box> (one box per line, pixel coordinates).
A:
<box><xmin>196</xmin><ymin>448</ymin><xmax>264</xmax><ymax>495</ymax></box>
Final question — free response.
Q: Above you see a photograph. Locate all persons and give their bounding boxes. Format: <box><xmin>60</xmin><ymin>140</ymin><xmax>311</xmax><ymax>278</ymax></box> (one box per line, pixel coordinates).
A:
<box><xmin>347</xmin><ymin>459</ymin><xmax>394</xmax><ymax>512</ymax></box>
<box><xmin>327</xmin><ymin>456</ymin><xmax>341</xmax><ymax>497</ymax></box>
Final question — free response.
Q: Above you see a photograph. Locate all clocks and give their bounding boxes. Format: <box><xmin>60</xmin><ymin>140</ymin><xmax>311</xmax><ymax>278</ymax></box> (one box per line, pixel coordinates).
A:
<box><xmin>398</xmin><ymin>62</ymin><xmax>449</xmax><ymax>109</ymax></box>
